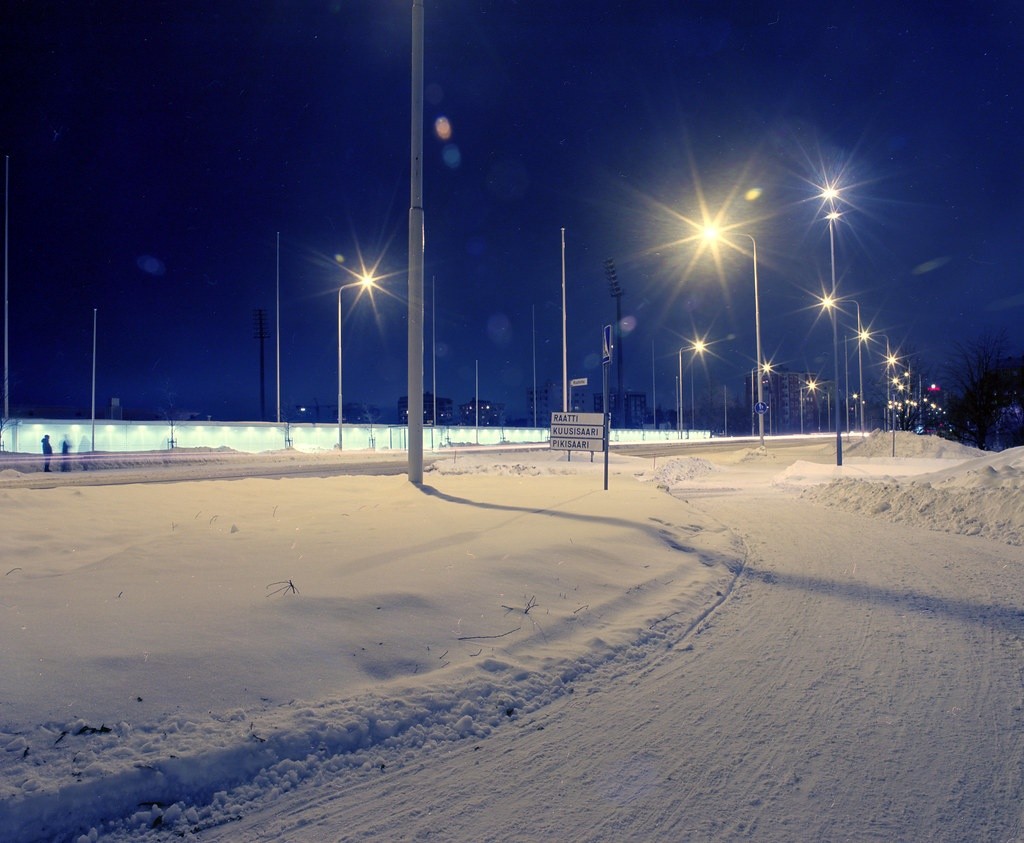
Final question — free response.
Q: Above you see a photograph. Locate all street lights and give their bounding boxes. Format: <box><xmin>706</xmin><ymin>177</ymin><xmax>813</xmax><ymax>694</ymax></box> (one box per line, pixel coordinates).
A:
<box><xmin>749</xmin><ymin>362</ymin><xmax>772</xmax><ymax>439</ymax></box>
<box><xmin>808</xmin><ymin>381</ymin><xmax>832</xmax><ymax>433</ymax></box>
<box><xmin>822</xmin><ymin>183</ymin><xmax>848</xmax><ymax>468</ymax></box>
<box><xmin>337</xmin><ymin>271</ymin><xmax>376</xmax><ymax>455</ymax></box>
<box><xmin>889</xmin><ymin>357</ymin><xmax>948</xmax><ymax>436</ymax></box>
<box><xmin>822</xmin><ymin>296</ymin><xmax>866</xmax><ymax>433</ymax></box>
<box><xmin>701</xmin><ymin>226</ymin><xmax>768</xmax><ymax>453</ymax></box>
<box><xmin>679</xmin><ymin>340</ymin><xmax>703</xmax><ymax>439</ymax></box>
<box><xmin>852</xmin><ymin>392</ymin><xmax>859</xmax><ymax>433</ymax></box>
<box><xmin>860</xmin><ymin>331</ymin><xmax>891</xmax><ymax>433</ymax></box>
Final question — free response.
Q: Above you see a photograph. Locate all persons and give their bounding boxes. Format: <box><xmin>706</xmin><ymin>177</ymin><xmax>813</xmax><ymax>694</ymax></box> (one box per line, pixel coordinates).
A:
<box><xmin>61</xmin><ymin>435</ymin><xmax>73</xmax><ymax>472</ymax></box>
<box><xmin>41</xmin><ymin>435</ymin><xmax>53</xmax><ymax>472</ymax></box>
